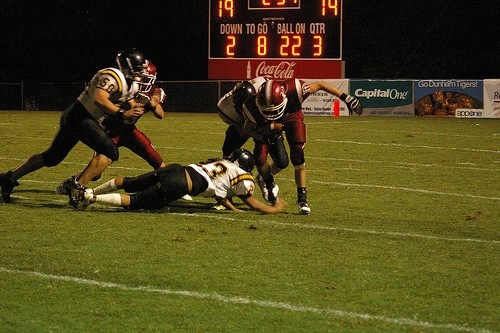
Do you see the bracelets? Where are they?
[151,107,156,112]
[340,93,348,101]
[117,108,125,118]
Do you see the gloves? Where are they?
[339,92,363,116]
[263,130,284,145]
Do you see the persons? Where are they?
[216,76,363,215]
[67,148,286,214]
[0,53,193,202]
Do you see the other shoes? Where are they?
[0,170,20,203]
[57,174,85,195]
[257,173,279,205]
[181,194,194,200]
[69,188,96,211]
[211,199,234,211]
[297,202,311,214]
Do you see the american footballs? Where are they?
[265,122,283,129]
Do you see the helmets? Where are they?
[133,59,157,92]
[228,148,255,172]
[115,48,146,82]
[256,80,288,120]
[232,80,259,124]
